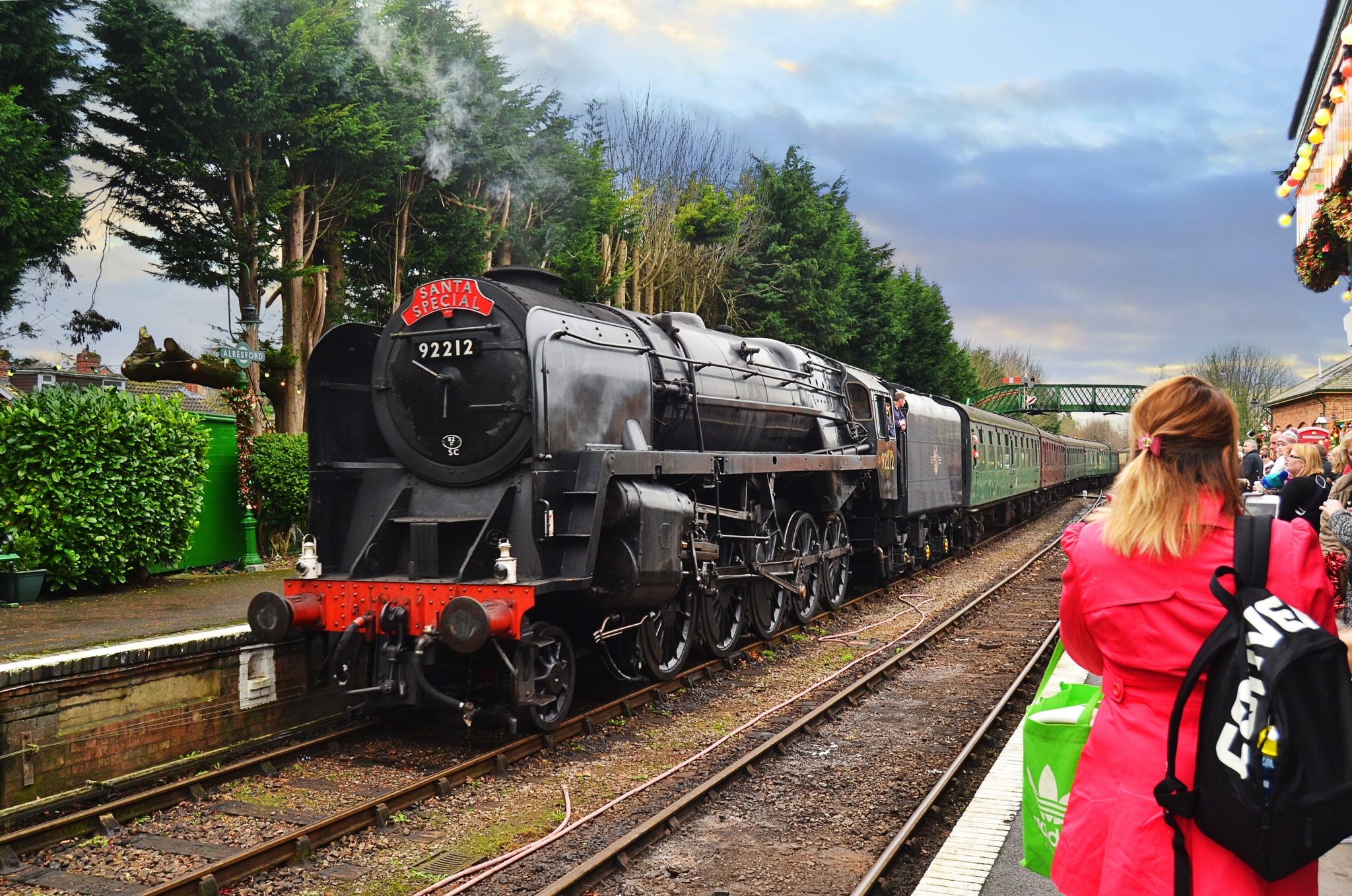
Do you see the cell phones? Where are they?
[1244,495,1281,519]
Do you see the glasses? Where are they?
[1289,454,1302,460]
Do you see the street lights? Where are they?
[1221,357,1230,397]
[1250,382,1267,444]
[228,262,265,570]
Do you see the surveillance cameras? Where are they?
[1247,430,1256,439]
[1021,376,1036,388]
[1250,397,1259,404]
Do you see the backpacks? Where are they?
[1152,516,1352,882]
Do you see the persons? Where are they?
[1253,428,1288,491]
[1270,427,1298,484]
[1264,443,1271,448]
[1261,451,1275,475]
[1259,447,1270,459]
[885,403,895,437]
[1315,430,1352,629]
[893,392,907,464]
[1261,471,1288,491]
[973,434,979,458]
[1278,443,1331,536]
[1237,440,1250,492]
[1050,375,1352,896]
[1239,439,1264,494]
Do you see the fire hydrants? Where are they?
[1331,398,1344,420]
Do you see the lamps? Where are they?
[1333,274,1351,300]
[1279,206,1296,226]
[1315,413,1329,424]
[1276,21,1352,198]
[1261,426,1271,431]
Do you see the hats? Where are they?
[1276,426,1298,456]
[1260,469,1288,489]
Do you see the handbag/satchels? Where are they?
[1020,679,1105,880]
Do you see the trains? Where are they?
[248,265,1119,736]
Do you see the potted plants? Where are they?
[0,529,47,605]
